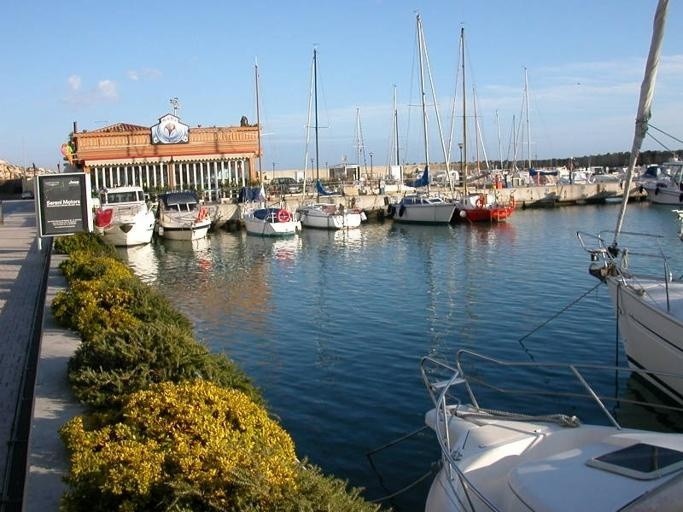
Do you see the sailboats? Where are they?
[577,0,682,413]
[387,12,459,224]
[441,25,517,223]
[298,45,368,230]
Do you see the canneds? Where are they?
[277,210,291,222]
[198,208,207,220]
[475,199,483,208]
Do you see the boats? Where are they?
[95,186,155,245]
[534,151,683,205]
[152,191,212,241]
[243,200,302,236]
[419,349,682,511]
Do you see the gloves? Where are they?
[534,151,683,205]
